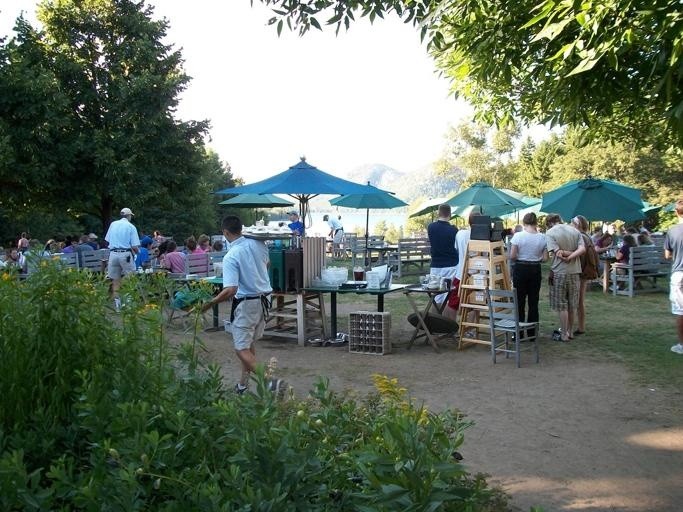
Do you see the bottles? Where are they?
[295,235,301,249]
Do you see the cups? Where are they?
[419,274,451,290]
[354,270,363,281]
[213,264,223,278]
[604,247,617,257]
[138,266,153,273]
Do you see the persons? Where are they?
[200,215,287,402]
[591,224,659,291]
[286,211,305,236]
[323,215,344,260]
[508,212,595,342]
[0,231,224,281]
[663,200,683,354]
[427,204,483,339]
[104,207,141,312]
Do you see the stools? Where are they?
[458,240,512,350]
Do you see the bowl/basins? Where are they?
[322,267,348,285]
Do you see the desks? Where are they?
[262,290,328,347]
[300,280,414,345]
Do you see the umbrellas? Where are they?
[217,192,295,226]
[408,175,663,231]
[219,155,396,236]
[329,179,410,248]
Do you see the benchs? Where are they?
[15,235,230,305]
[612,235,673,298]
[326,233,433,278]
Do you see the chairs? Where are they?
[485,286,539,368]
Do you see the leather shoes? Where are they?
[511,333,535,341]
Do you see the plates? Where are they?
[243,226,292,235]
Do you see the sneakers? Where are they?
[671,343,683,354]
[269,379,277,391]
[234,384,248,394]
[115,304,122,313]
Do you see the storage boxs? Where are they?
[470,216,504,242]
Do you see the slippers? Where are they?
[553,327,584,341]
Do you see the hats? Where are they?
[88,233,98,239]
[142,237,153,244]
[120,208,135,216]
